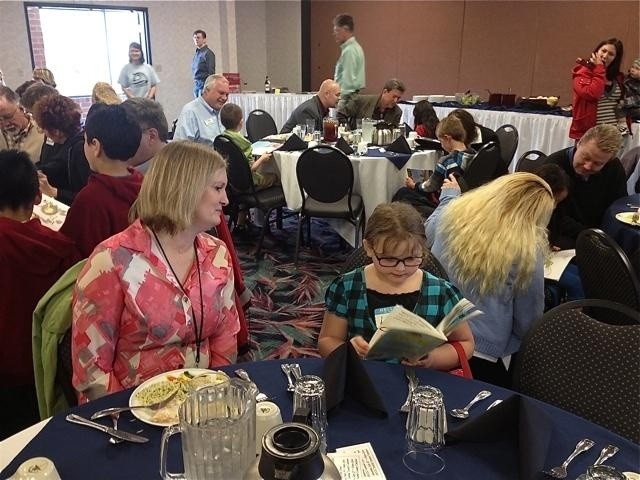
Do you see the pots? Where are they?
[488,93,518,110]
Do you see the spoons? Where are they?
[576,444,621,480]
[282,363,296,391]
[449,390,492,419]
[108,412,124,444]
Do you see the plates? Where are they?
[413,94,457,104]
[614,210,640,226]
[130,368,231,429]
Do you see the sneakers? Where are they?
[616,121,630,136]
[231,224,278,247]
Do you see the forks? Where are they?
[539,438,597,479]
[90,389,180,420]
[235,368,277,403]
[400,377,420,413]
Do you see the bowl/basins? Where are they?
[519,94,561,111]
[455,92,480,107]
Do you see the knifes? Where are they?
[66,414,150,443]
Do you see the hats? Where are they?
[33,68,55,87]
[633,57,640,66]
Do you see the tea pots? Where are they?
[242,407,342,480]
[158,377,259,480]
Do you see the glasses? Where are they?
[371,246,425,267]
[0,107,17,123]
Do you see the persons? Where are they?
[568,39,639,147]
[426,124,628,388]
[0,76,171,407]
[329,13,365,128]
[172,73,279,191]
[119,42,160,103]
[315,202,474,381]
[280,77,499,204]
[191,30,215,100]
[70,142,240,407]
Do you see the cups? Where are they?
[291,116,423,157]
[190,369,231,421]
[292,375,329,439]
[402,385,447,475]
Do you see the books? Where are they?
[363,298,484,366]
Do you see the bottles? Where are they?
[264,75,271,93]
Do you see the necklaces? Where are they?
[151,224,204,369]
